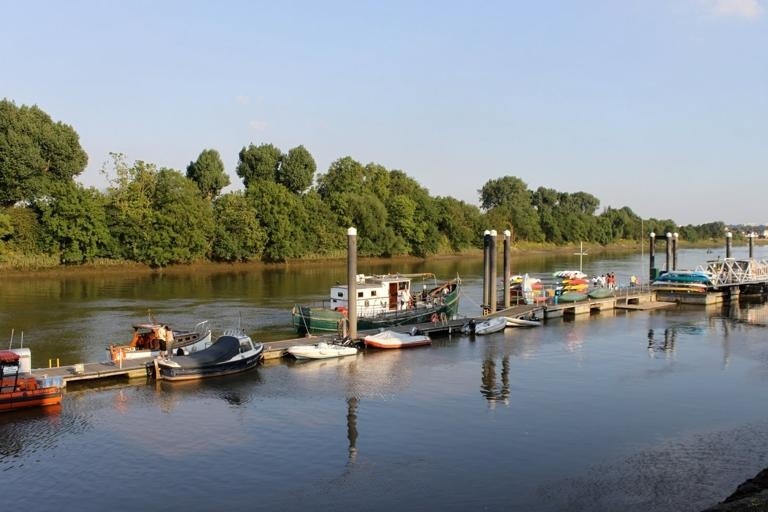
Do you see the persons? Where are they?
[140,337,145,350]
[592,271,616,291]
[630,274,637,287]
[400,284,413,310]
[155,323,175,358]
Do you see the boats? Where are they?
[496,275,549,305]
[292,272,461,335]
[145,334,264,382]
[547,269,613,302]
[284,343,359,360]
[106,319,211,363]
[0,348,62,413]
[460,316,507,336]
[360,331,432,350]
[500,313,542,328]
[650,257,755,292]
